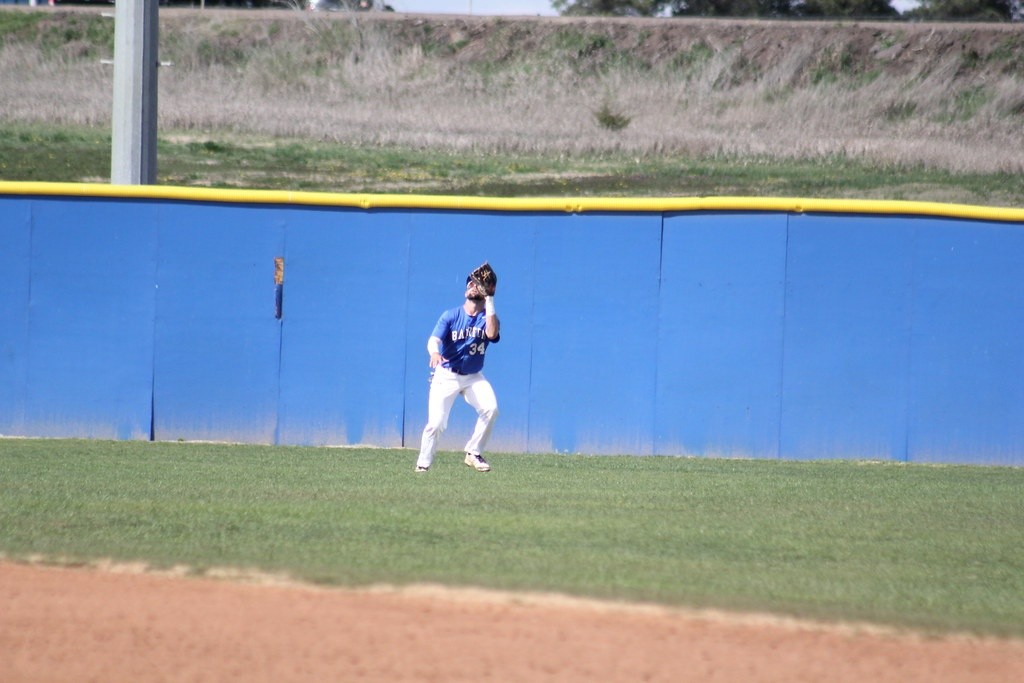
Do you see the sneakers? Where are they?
[414,466,428,472]
[464,453,490,472]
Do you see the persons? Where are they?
[414,261,501,472]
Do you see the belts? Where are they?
[449,367,467,375]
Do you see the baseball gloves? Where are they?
[470,262,498,296]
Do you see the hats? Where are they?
[466,275,473,286]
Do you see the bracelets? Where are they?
[427,341,438,356]
[486,296,496,316]
